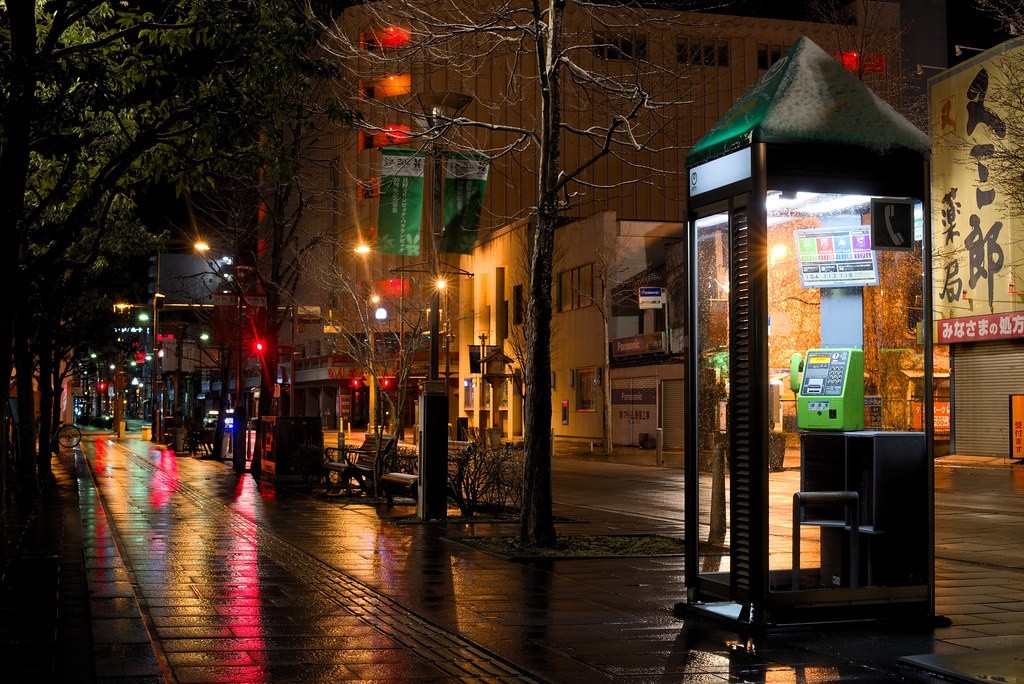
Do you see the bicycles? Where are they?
[34,412,82,448]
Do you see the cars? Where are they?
[74,406,82,415]
[208,409,234,428]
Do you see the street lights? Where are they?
[436,277,451,397]
[194,240,237,263]
[91,314,210,370]
[354,245,405,440]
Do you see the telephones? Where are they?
[788,348,865,430]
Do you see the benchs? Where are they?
[181,428,217,455]
[324,434,393,497]
[380,441,475,514]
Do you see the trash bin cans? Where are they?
[141,425,152,441]
[638,432,648,448]
[770,432,786,471]
[488,427,502,451]
[172,428,187,452]
[212,431,229,459]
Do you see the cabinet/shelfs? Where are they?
[799,429,927,590]
